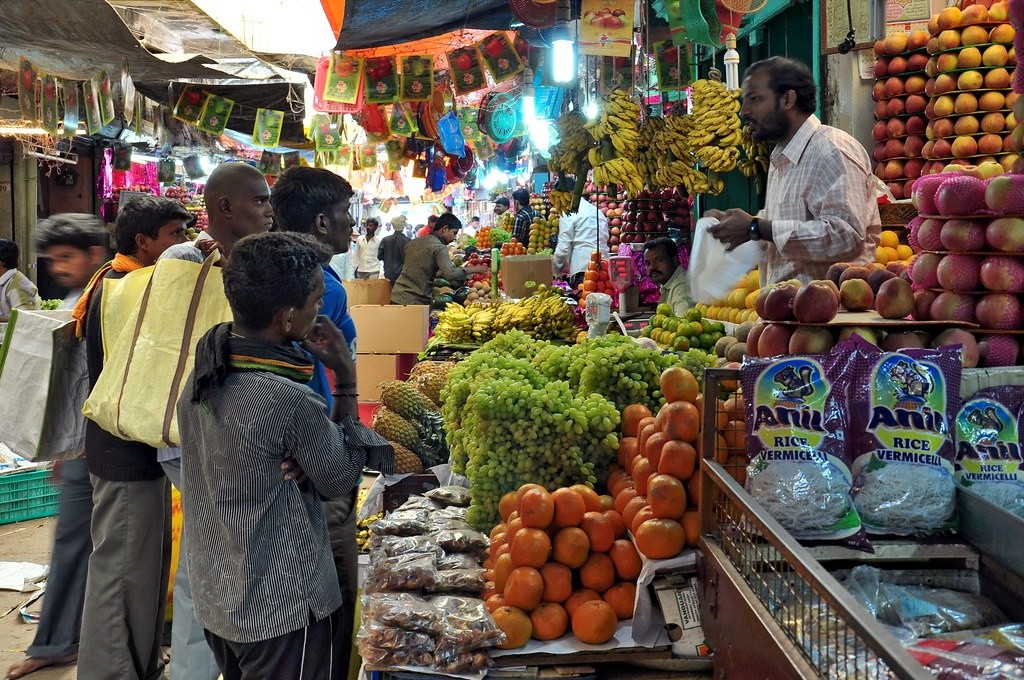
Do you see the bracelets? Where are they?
[334,381,357,389]
[332,393,359,396]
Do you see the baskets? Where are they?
[0,469,63,525]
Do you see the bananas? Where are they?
[427,288,583,346]
[547,79,772,215]
[355,487,384,553]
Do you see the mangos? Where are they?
[696,270,764,325]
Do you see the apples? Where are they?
[585,6,627,29]
[109,185,208,240]
[526,196,558,255]
[747,261,926,356]
[908,173,1024,368]
[871,0,1024,198]
[334,39,501,81]
[502,212,513,232]
[582,181,691,253]
[463,252,503,290]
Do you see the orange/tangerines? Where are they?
[607,365,754,559]
[480,481,643,648]
[641,302,726,353]
[474,225,491,249]
[578,251,616,308]
[875,230,915,265]
[500,236,527,257]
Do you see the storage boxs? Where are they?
[499,254,552,301]
[339,274,432,432]
[0,463,63,526]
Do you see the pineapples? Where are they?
[372,361,454,475]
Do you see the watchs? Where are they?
[750,218,760,241]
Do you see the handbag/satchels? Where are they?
[81,246,235,448]
[1,263,110,463]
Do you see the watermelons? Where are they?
[432,263,470,309]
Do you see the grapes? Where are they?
[40,298,63,309]
[488,228,512,243]
[439,328,722,529]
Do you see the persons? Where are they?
[1,57,882,680]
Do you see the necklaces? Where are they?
[228,329,246,339]
